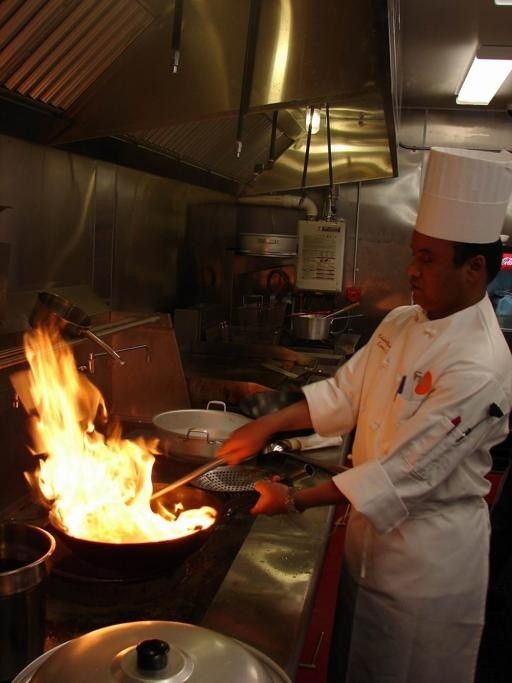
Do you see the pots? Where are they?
[27,287,129,371]
[2,519,55,665]
[54,478,302,556]
[154,399,255,460]
[286,301,359,339]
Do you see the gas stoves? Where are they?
[10,417,289,651]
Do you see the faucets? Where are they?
[265,268,294,305]
[89,343,151,364]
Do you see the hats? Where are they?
[413,147,512,245]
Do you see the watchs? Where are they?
[285,484,306,516]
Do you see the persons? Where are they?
[216,228,512,682]
